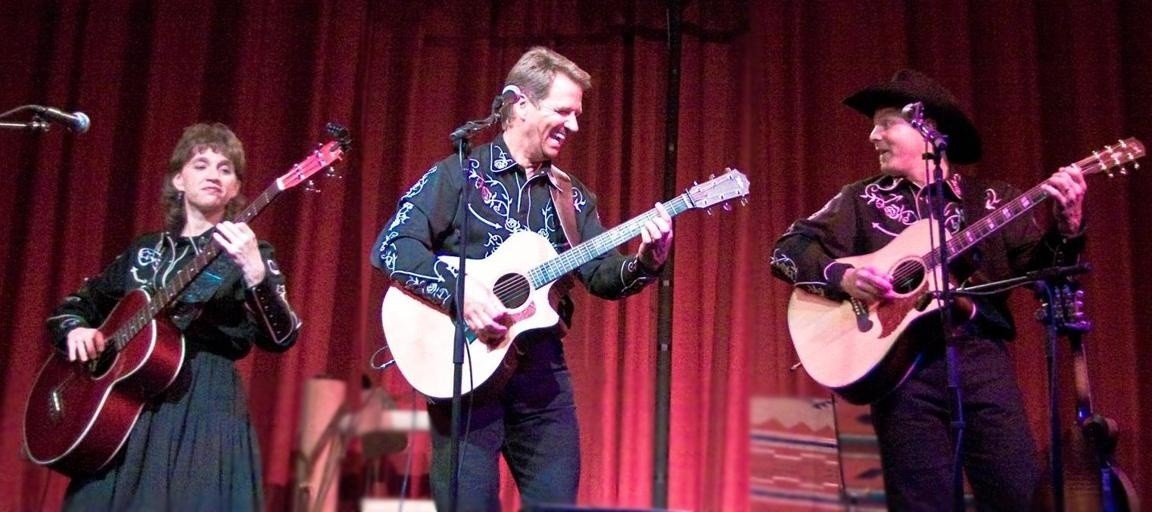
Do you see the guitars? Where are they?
[787,137,1146,406]
[380,167,751,410]
[20,122,353,477]
[1060,290,1139,512]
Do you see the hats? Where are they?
[841,69,983,165]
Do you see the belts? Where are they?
[943,318,991,340]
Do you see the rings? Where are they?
[1063,186,1071,194]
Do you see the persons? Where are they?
[769,67,1088,512]
[45,121,304,512]
[369,43,674,512]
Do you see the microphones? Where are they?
[445,94,507,141]
[900,98,947,158]
[29,98,92,137]
